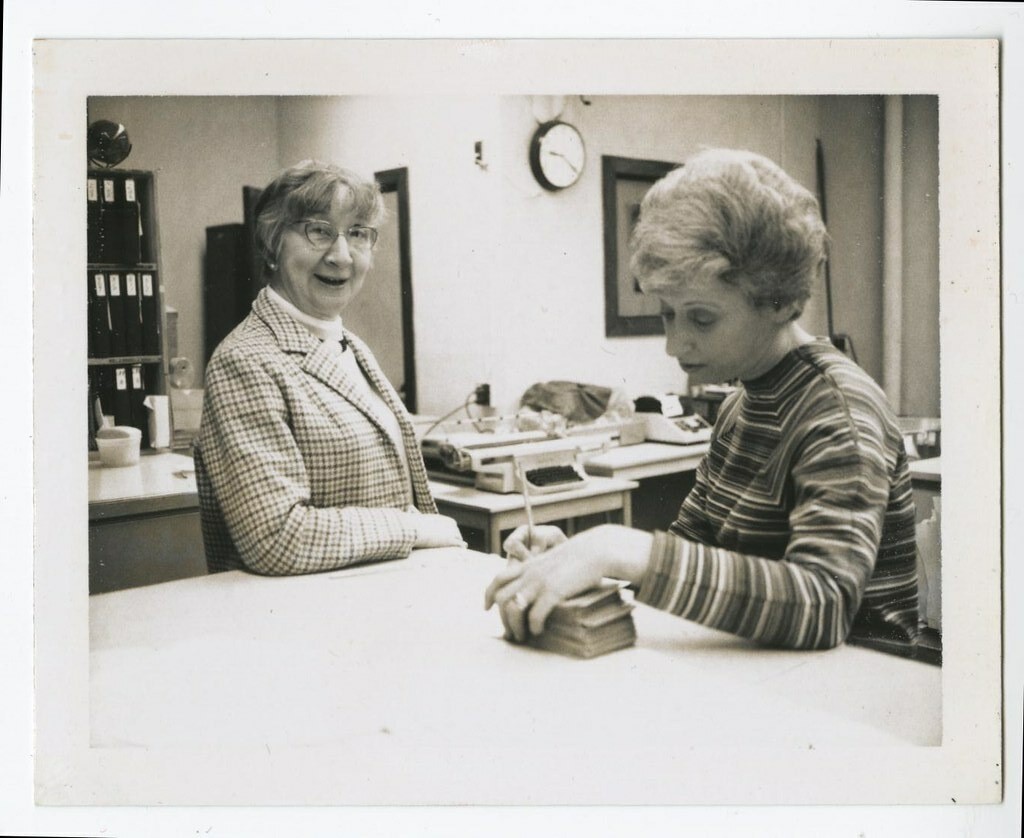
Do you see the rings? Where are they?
[513,594,527,611]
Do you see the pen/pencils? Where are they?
[516,459,538,551]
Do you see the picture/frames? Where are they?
[602,154,686,336]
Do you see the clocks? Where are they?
[530,119,585,191]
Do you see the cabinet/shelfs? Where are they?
[86,164,175,453]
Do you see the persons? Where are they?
[483,149,920,653]
[193,159,469,577]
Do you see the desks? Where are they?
[89,448,209,596]
[428,437,713,554]
[89,537,942,745]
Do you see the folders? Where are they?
[88,363,152,451]
[86,174,142,265]
[88,271,161,358]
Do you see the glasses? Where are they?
[287,219,378,249]
